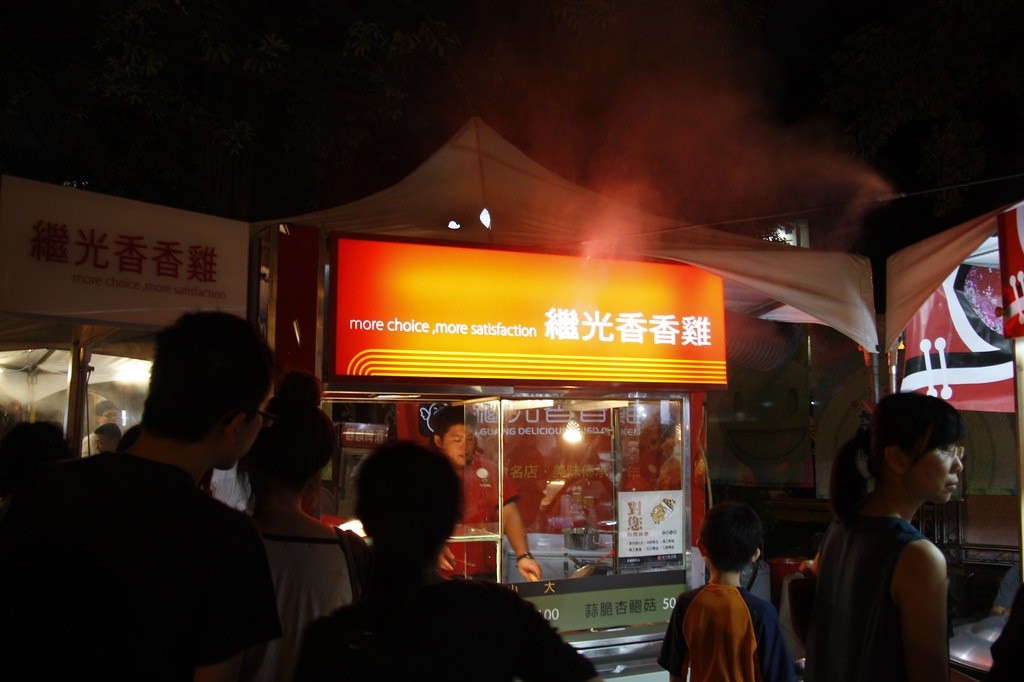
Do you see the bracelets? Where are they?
[517,553,533,562]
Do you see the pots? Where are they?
[562,527,618,551]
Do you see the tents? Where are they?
[69,104,881,411]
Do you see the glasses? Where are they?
[223,407,276,427]
[100,413,120,420]
[931,444,965,459]
[94,439,109,445]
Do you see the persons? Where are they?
[428,405,542,582]
[657,498,795,682]
[94,423,123,453]
[81,400,120,458]
[801,391,964,682]
[0,311,600,682]
